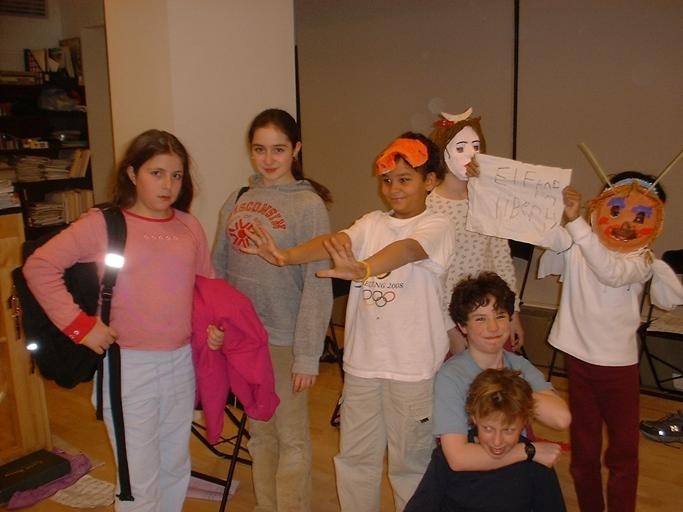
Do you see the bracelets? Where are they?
[521,439,538,465]
[352,260,372,284]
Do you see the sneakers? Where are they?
[639,408,682,443]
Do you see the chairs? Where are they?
[189,392,253,512]
[639,249,683,403]
[505,241,534,361]
[330,279,350,428]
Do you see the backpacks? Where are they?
[7,201,127,388]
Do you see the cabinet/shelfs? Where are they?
[0,28,117,468]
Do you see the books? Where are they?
[1,35,96,234]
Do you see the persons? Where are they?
[204,104,340,512]
[235,131,459,511]
[461,147,682,511]
[426,268,576,477]
[20,126,229,511]
[421,104,528,363]
[402,365,571,509]
